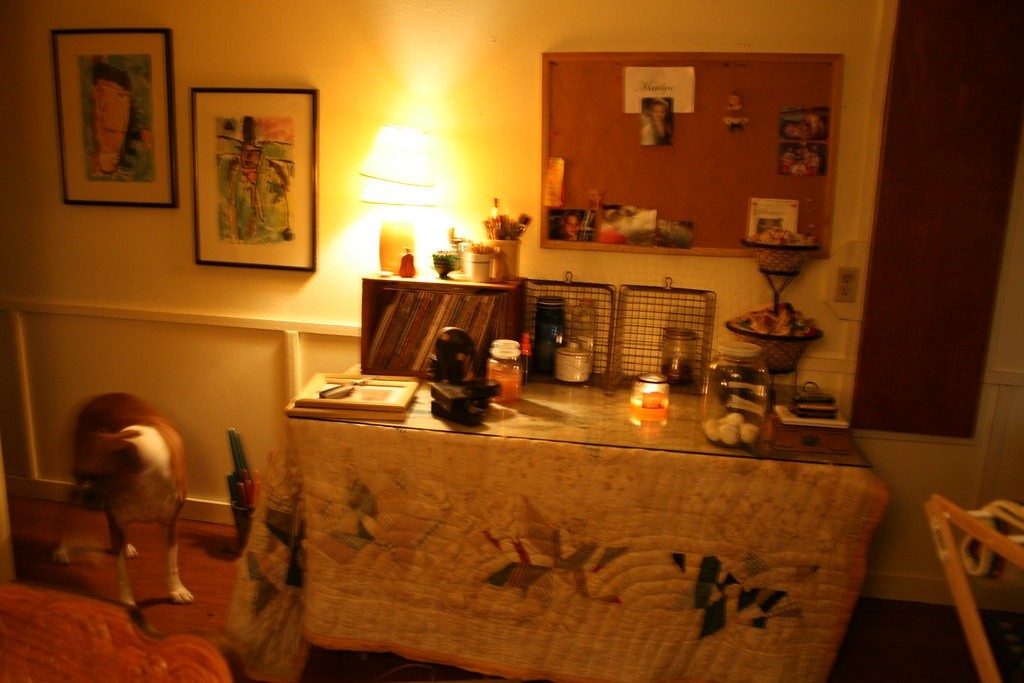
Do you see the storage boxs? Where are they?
[766,404,851,455]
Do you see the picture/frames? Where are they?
[190,87,319,272]
[50,26,182,209]
[293,373,420,413]
[284,388,408,421]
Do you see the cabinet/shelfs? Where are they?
[361,272,529,380]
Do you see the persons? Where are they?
[643,98,672,144]
[779,112,827,177]
[561,212,580,242]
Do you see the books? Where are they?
[368,289,504,376]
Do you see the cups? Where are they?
[431,250,463,280]
[554,338,594,383]
[630,372,670,423]
[481,238,523,282]
[464,252,491,283]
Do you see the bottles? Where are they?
[660,326,698,387]
[533,293,566,378]
[399,247,417,279]
[486,338,522,406]
[700,340,769,450]
[570,297,595,376]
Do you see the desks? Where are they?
[286,359,874,683]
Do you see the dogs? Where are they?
[46,392,194,611]
